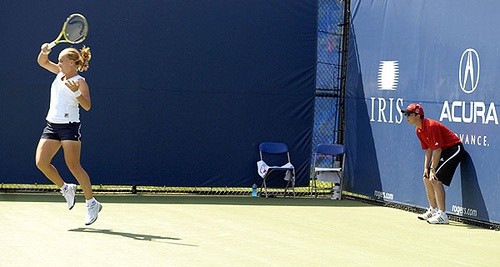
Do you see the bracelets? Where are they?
[40,50,52,55]
[71,89,82,99]
[429,167,434,169]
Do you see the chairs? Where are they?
[258,142,295,199]
[310,143,345,200]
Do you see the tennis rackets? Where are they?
[42,13,88,53]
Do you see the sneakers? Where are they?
[59,184,77,210]
[84,200,102,225]
[426,212,449,224]
[417,210,438,221]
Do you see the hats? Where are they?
[401,103,424,116]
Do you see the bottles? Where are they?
[334,183,340,200]
[252,183,257,196]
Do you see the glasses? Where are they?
[404,113,416,116]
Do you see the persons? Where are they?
[32,42,103,226]
[399,102,465,225]
